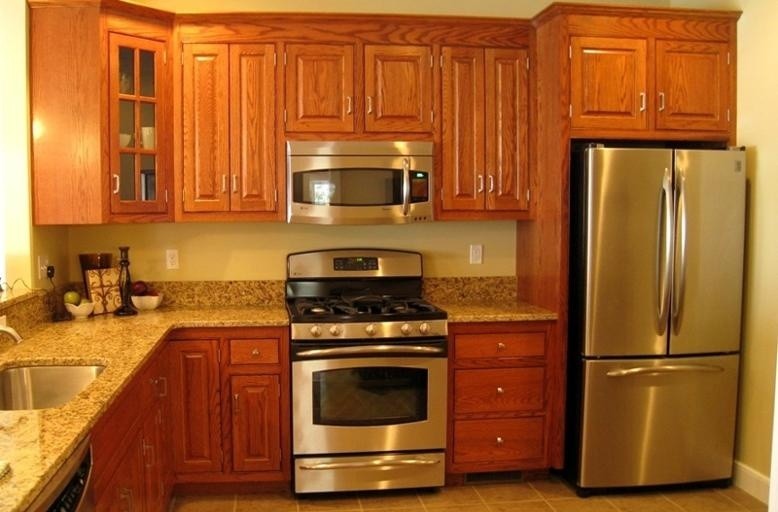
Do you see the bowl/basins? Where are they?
[64,303,96,320]
[130,294,164,312]
[118,133,132,148]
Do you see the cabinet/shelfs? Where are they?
[532,1,744,142]
[170,43,288,226]
[170,330,294,500]
[27,1,174,229]
[287,43,431,146]
[92,379,140,511]
[432,43,531,222]
[446,323,554,487]
[138,324,178,512]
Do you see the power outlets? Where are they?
[37,255,50,281]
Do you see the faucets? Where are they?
[1,326,23,346]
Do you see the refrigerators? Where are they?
[568,143,745,497]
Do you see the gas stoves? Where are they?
[292,295,441,316]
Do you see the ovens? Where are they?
[290,335,448,498]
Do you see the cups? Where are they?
[133,125,155,148]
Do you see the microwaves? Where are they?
[285,140,434,227]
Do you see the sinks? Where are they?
[1,364,106,412]
